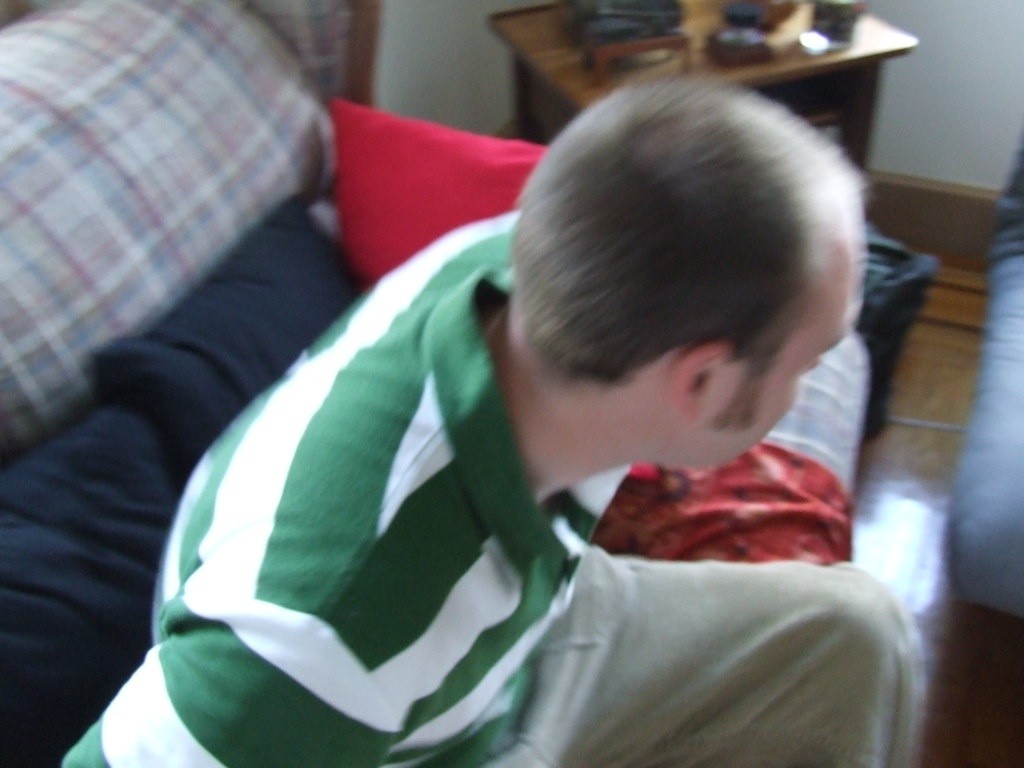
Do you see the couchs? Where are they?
[0,0,870,768]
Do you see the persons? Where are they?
[63,77,924,768]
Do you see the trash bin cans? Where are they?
[856,231,938,439]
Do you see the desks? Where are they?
[487,0,918,146]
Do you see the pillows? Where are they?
[87,192,359,469]
[0,405,185,768]
[329,101,544,282]
